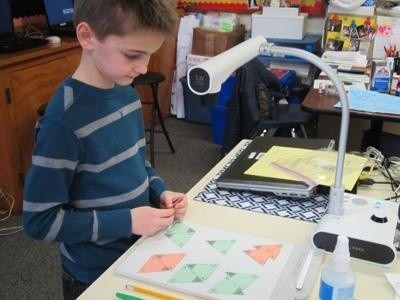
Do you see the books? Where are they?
[313,50,373,92]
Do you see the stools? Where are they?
[130,71,174,166]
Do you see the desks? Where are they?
[76,138,400,295]
[299,55,400,159]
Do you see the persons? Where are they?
[22,0,189,300]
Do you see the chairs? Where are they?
[233,56,316,139]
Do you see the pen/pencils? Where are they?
[384,45,399,57]
[115,285,181,300]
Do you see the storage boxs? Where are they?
[180,23,245,124]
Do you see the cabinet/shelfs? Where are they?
[0,30,174,212]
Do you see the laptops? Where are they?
[216,135,336,199]
